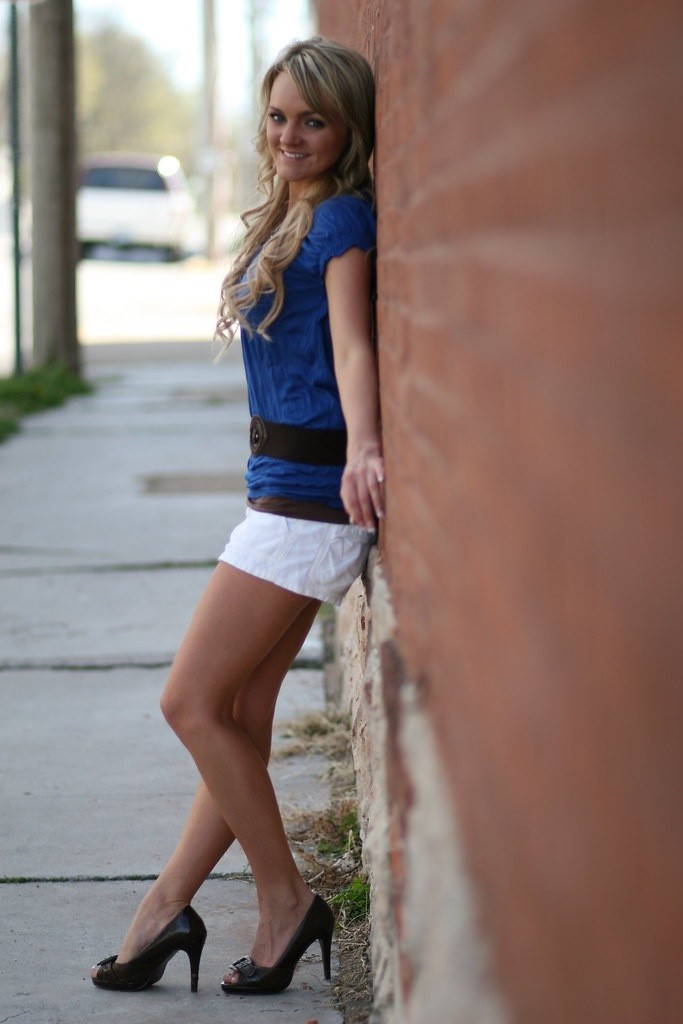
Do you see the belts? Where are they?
[247,415,352,469]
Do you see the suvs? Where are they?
[71,152,204,262]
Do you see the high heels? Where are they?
[93,907,209,993]
[218,893,336,994]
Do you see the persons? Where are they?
[88,36,386,996]
